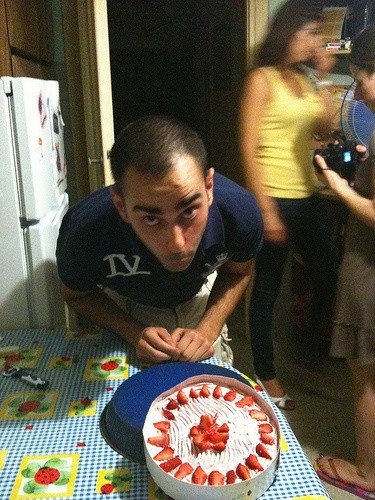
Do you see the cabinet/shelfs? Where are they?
[0,0,59,80]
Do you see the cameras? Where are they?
[313,130,360,171]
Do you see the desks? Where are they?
[0,324,334,500]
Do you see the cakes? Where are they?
[142,380,278,486]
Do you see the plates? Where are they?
[98,360,256,467]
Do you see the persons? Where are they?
[239,0,374,500]
[55,115,263,364]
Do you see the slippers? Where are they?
[313,452,375,500]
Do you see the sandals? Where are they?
[270,390,296,410]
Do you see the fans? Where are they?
[341,77,375,151]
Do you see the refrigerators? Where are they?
[1,74,71,335]
